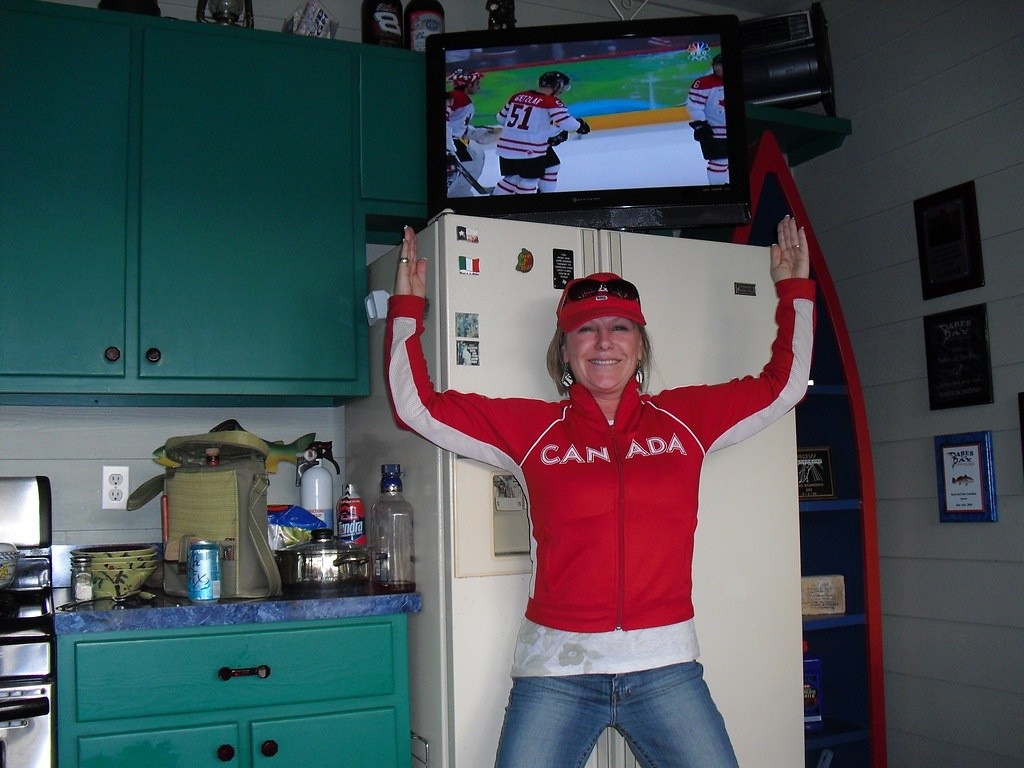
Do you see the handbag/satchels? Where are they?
[164,430,273,599]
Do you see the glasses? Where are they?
[561,278,641,310]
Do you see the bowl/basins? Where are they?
[70,546,159,599]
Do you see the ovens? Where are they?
[0,679,58,767]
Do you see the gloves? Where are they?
[574,118,590,135]
[462,124,499,145]
[547,129,568,147]
[689,119,715,144]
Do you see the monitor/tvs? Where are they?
[426,13,752,232]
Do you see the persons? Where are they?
[490,71,591,195]
[383,214,816,768]
[446,106,461,197]
[446,68,503,197]
[686,53,731,185]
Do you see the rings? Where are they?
[792,244,800,249]
[399,258,408,263]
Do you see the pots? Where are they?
[0,544,26,591]
[273,530,387,599]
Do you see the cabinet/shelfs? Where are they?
[0,0,431,408]
[730,128,889,768]
[53,586,422,768]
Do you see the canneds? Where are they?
[186,540,222,604]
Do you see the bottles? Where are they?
[73,556,93,606]
[337,484,367,546]
[368,463,416,592]
[206,448,219,466]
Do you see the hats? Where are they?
[556,272,647,333]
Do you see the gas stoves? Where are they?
[0,476,53,678]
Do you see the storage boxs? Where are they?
[802,575,845,615]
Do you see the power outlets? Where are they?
[102,465,130,511]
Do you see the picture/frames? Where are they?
[933,430,1000,523]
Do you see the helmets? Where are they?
[712,53,722,77]
[452,66,484,86]
[539,71,572,95]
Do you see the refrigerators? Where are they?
[343,206,805,767]
